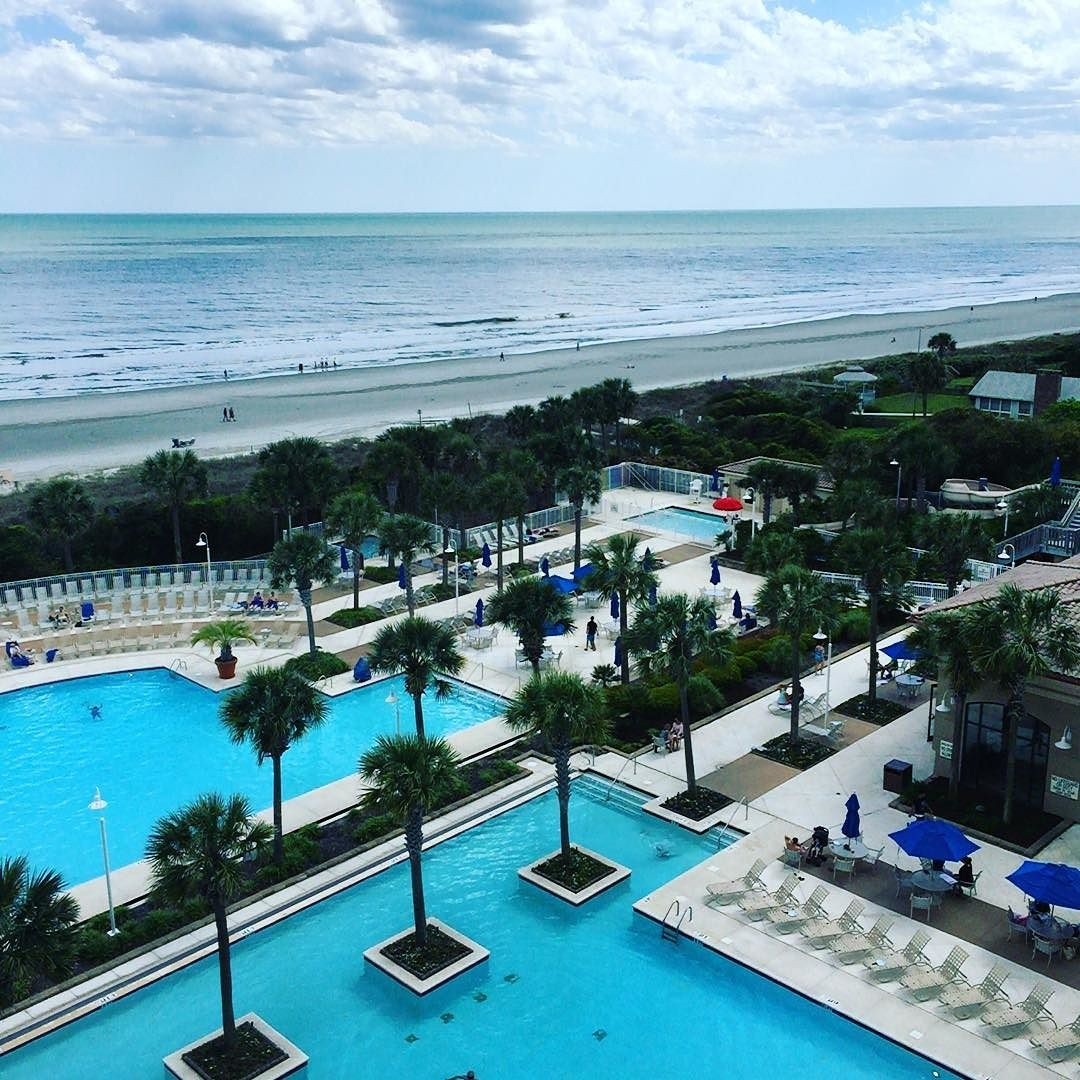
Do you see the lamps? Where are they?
[935,690,951,713]
[1055,725,1072,750]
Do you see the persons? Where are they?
[660,718,684,753]
[814,640,825,675]
[952,856,973,896]
[790,838,823,858]
[10,644,25,659]
[55,606,71,626]
[1029,897,1050,921]
[584,616,598,651]
[249,591,278,610]
[875,652,898,679]
[692,475,703,504]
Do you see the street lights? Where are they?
[742,487,756,542]
[195,532,216,619]
[889,458,904,526]
[444,538,459,619]
[385,684,400,737]
[87,785,121,937]
[996,492,1009,539]
[811,622,833,732]
[997,544,1016,570]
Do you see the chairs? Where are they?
[705,815,1080,1063]
[863,657,880,678]
[893,659,908,677]
[330,521,765,673]
[803,688,834,715]
[0,567,290,672]
[800,721,842,743]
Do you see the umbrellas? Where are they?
[878,641,926,680]
[1048,456,1061,491]
[1005,860,1080,920]
[713,497,743,526]
[341,543,350,578]
[399,561,407,607]
[475,542,743,683]
[842,791,860,848]
[888,819,981,878]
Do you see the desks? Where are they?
[895,674,925,695]
[912,868,956,892]
[828,837,869,859]
[1027,914,1075,943]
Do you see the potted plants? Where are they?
[190,617,258,679]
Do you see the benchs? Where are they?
[769,695,812,717]
